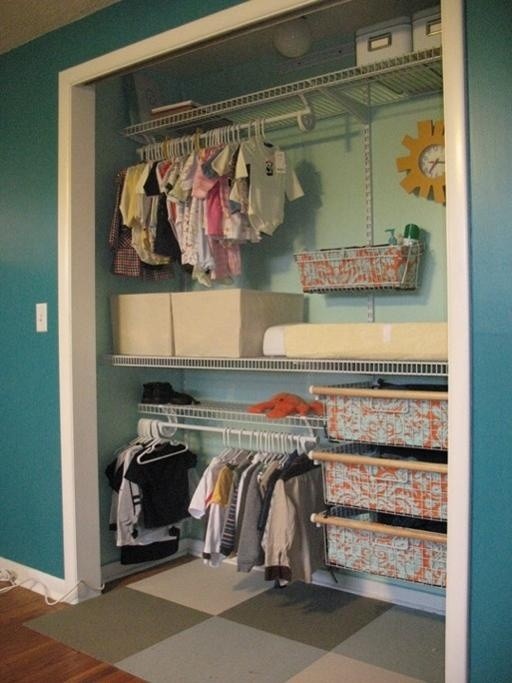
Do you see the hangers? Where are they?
[136,115,273,162]
[133,420,323,472]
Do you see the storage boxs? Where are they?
[413,5,445,73]
[110,293,171,358]
[172,288,306,356]
[352,12,441,94]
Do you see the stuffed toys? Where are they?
[247,392,325,418]
